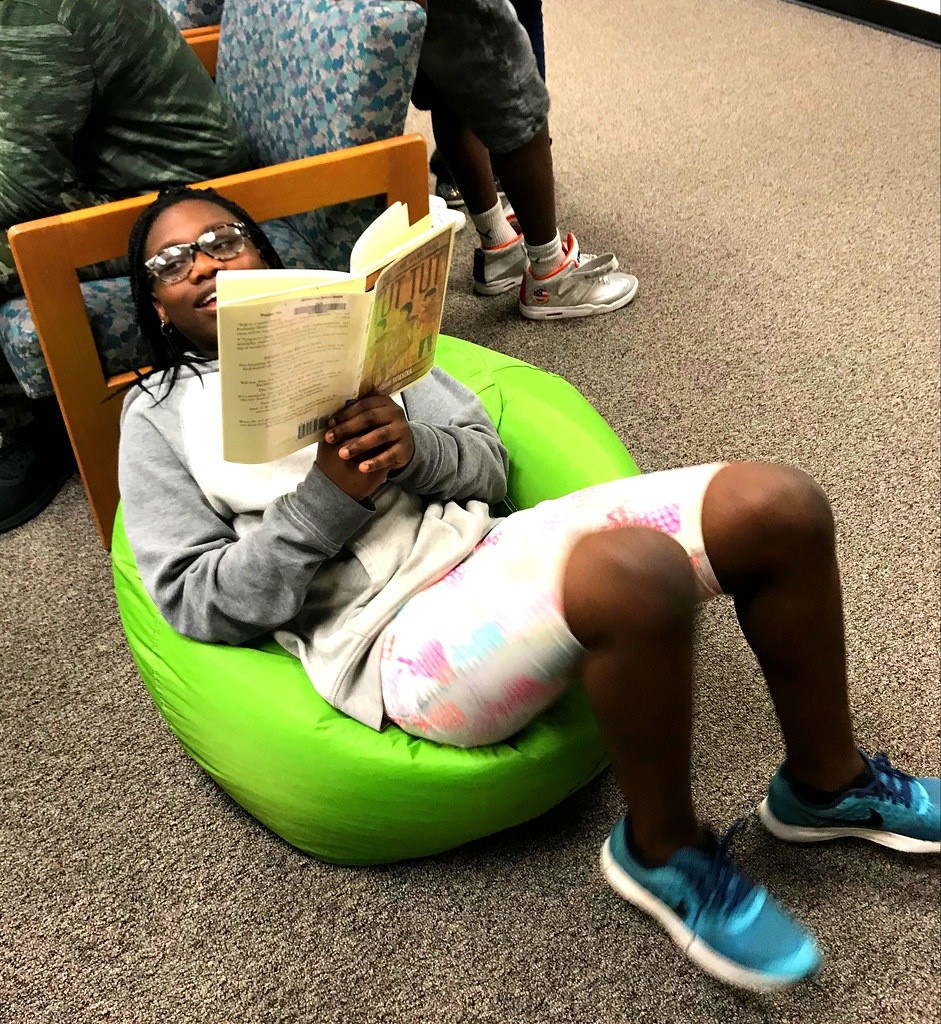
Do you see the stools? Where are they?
[111,341,648,867]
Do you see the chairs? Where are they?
[0,0,428,539]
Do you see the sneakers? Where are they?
[519,233,640,319]
[760,750,941,851]
[599,816,823,991]
[473,215,530,295]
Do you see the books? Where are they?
[216,202,455,462]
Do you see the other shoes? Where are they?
[435,177,505,206]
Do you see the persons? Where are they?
[99,186,941,989]
[0,0,639,536]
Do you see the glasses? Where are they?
[144,222,248,283]
